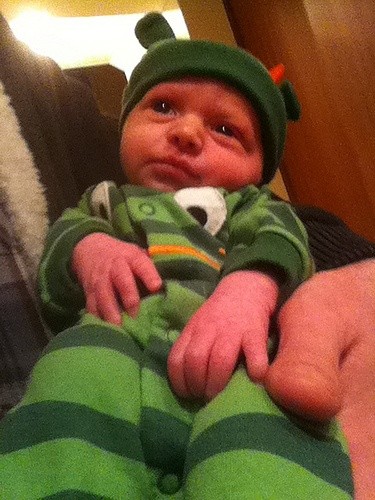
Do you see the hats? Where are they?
[118,11,300,186]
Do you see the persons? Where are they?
[0,12,354,500]
[0,12,375,500]
